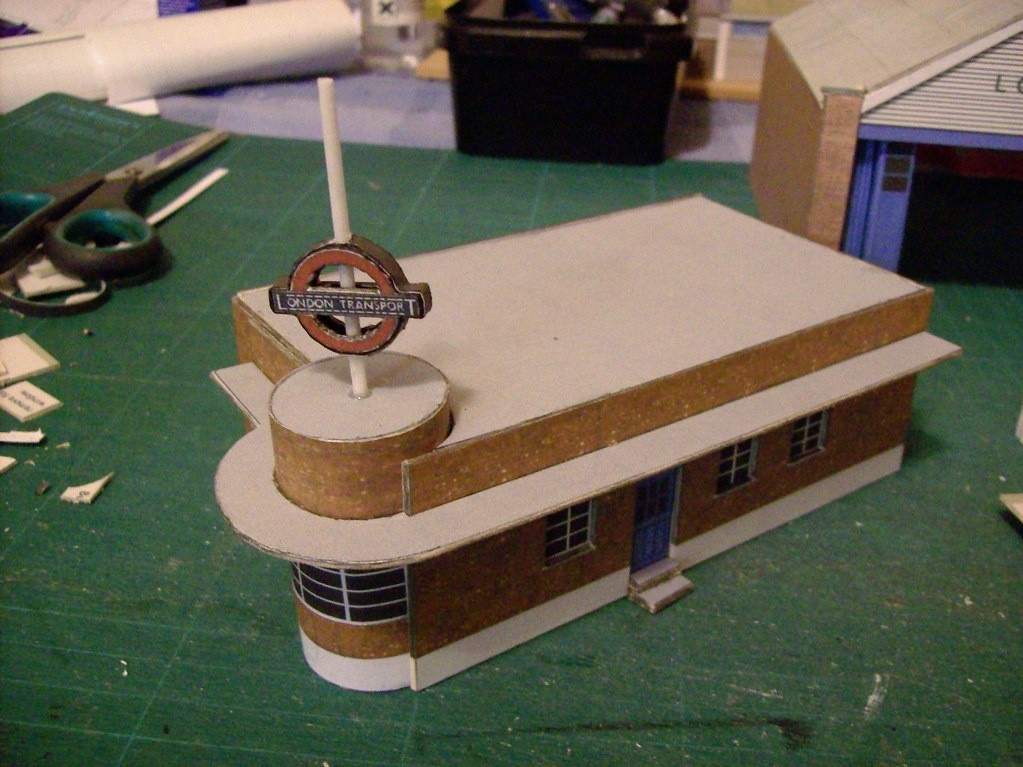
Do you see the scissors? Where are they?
[0,129,231,281]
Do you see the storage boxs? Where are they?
[436,1,694,166]
